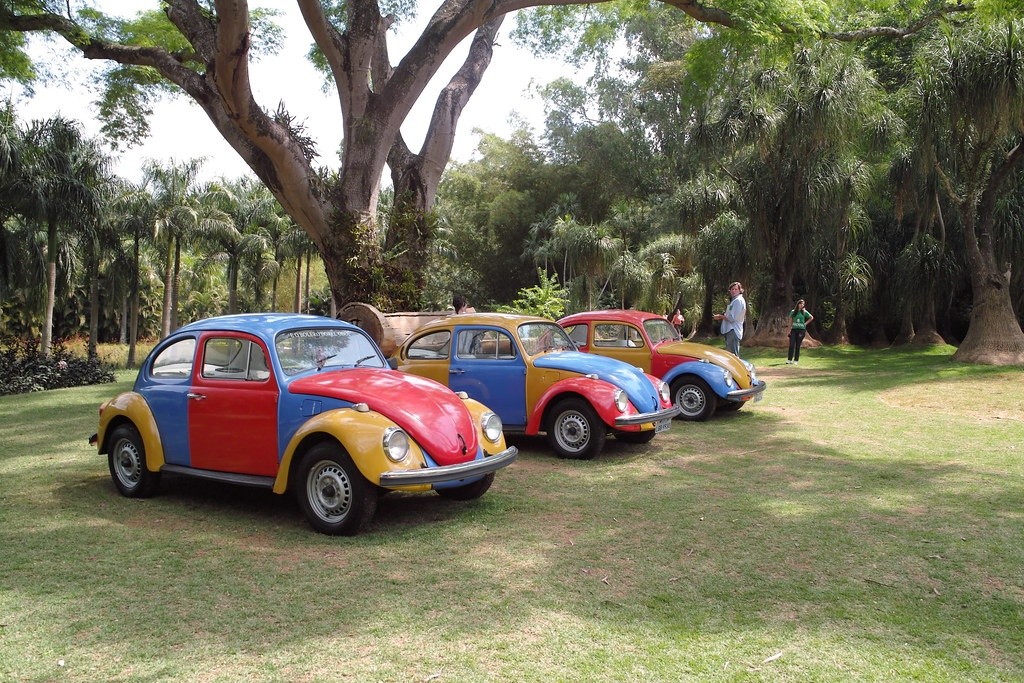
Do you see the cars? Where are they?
[89,311,520,538]
[530,309,766,421]
[383,312,680,459]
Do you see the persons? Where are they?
[671,309,684,335]
[712,283,746,356]
[787,300,813,365]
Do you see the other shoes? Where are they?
[793,361,798,365]
[786,360,791,364]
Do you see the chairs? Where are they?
[616,328,636,348]
